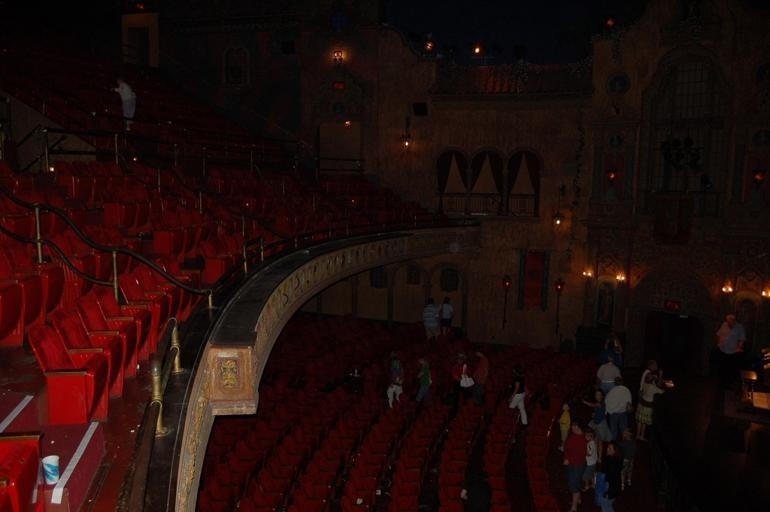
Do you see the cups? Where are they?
[43,454,61,485]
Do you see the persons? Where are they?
[107,78,137,134]
[714,313,746,392]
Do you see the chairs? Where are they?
[0,147,439,511]
[207,322,598,510]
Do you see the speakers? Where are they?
[407,264,420,284]
[282,41,294,54]
[371,267,387,287]
[439,269,458,292]
[413,102,427,116]
